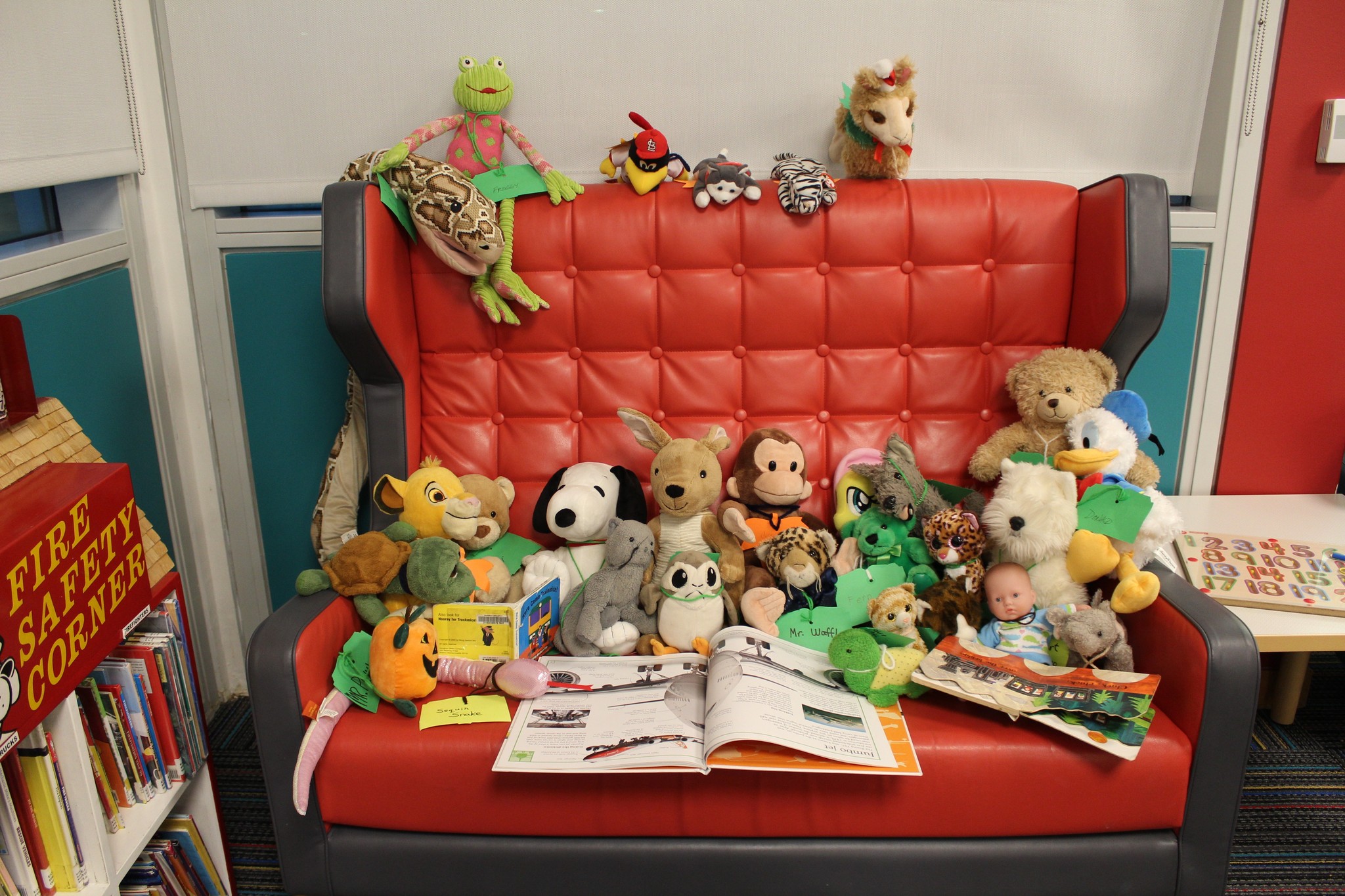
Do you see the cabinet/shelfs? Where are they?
[2,572,235,896]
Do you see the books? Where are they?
[0,596,222,896]
[430,580,1158,775]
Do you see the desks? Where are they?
[1160,493,1345,725]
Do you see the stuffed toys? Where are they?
[338,58,917,325]
[296,347,1182,676]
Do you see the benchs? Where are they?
[245,175,1263,896]
